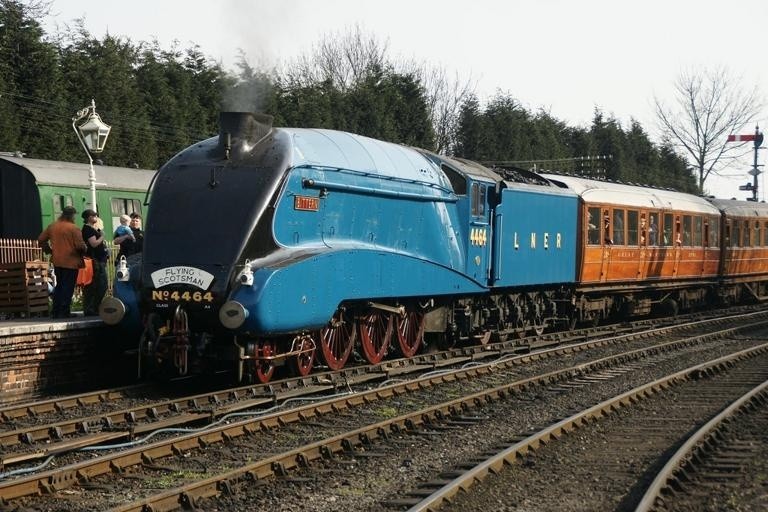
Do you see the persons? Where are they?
[113,211,144,265]
[92,211,110,260]
[38,204,88,319]
[81,209,109,317]
[112,213,136,268]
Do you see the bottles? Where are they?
[82,210,98,218]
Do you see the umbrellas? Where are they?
[53,311,78,318]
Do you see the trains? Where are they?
[134,110,768,388]
[1,151,160,311]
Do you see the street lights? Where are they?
[70,97,112,229]
